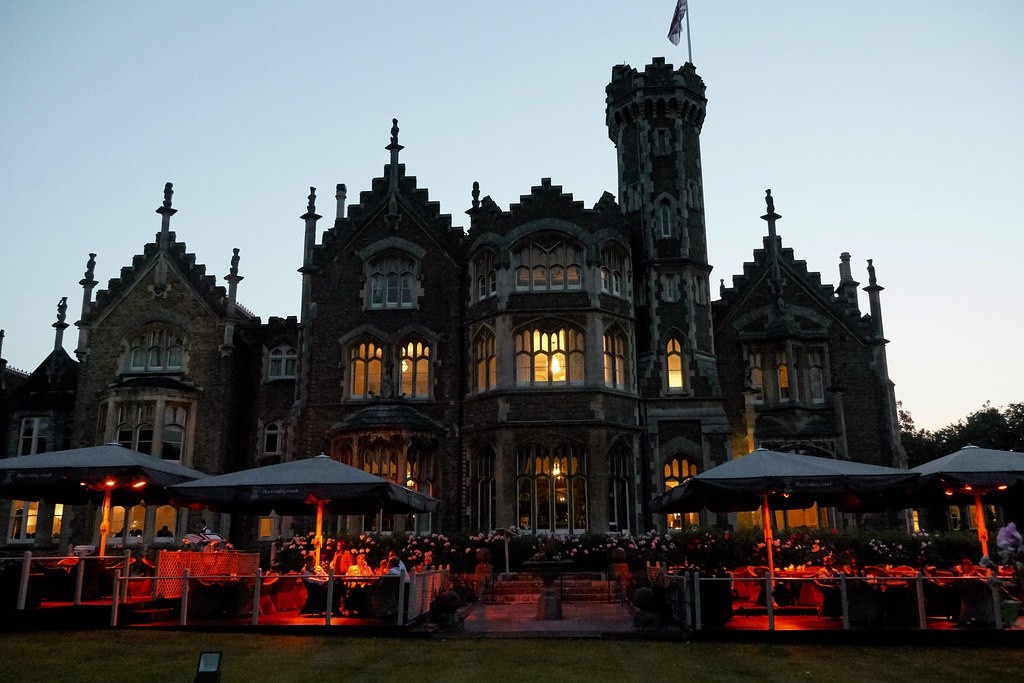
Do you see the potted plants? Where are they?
[474,550,494,576]
[609,551,630,577]
[533,562,562,622]
[436,590,460,630]
[629,588,661,630]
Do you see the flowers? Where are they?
[526,542,570,562]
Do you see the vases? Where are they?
[522,560,574,576]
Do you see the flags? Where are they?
[666,0,686,46]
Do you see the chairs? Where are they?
[189,572,400,615]
[28,558,157,599]
[733,566,1016,605]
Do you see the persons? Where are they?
[330,537,356,576]
[114,524,143,537]
[814,555,843,617]
[996,522,1024,564]
[610,548,634,595]
[377,550,411,586]
[157,526,173,537]
[961,559,986,578]
[198,519,212,534]
[342,553,375,607]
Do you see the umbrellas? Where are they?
[649,445,922,578]
[0,443,215,561]
[169,450,438,569]
[907,442,1024,556]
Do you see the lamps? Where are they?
[195,650,223,683]
